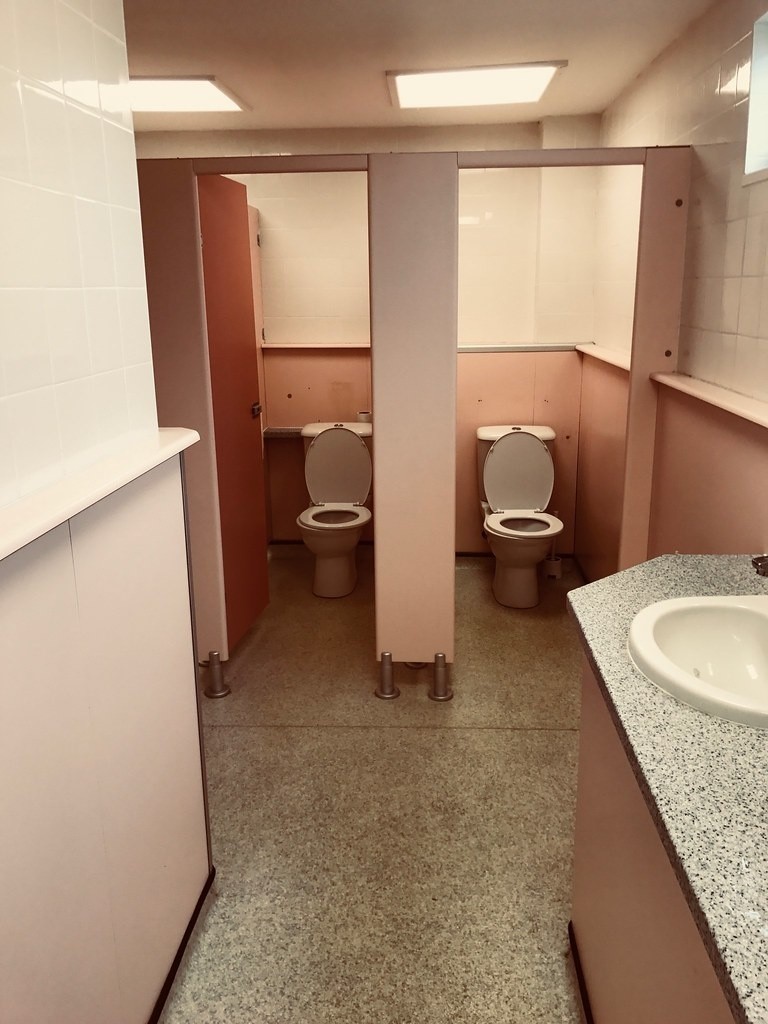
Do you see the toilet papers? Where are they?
[356,410,372,423]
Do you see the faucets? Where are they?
[752,555,768,576]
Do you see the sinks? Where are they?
[625,593,768,731]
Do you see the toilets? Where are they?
[476,425,557,609]
[296,422,373,598]
